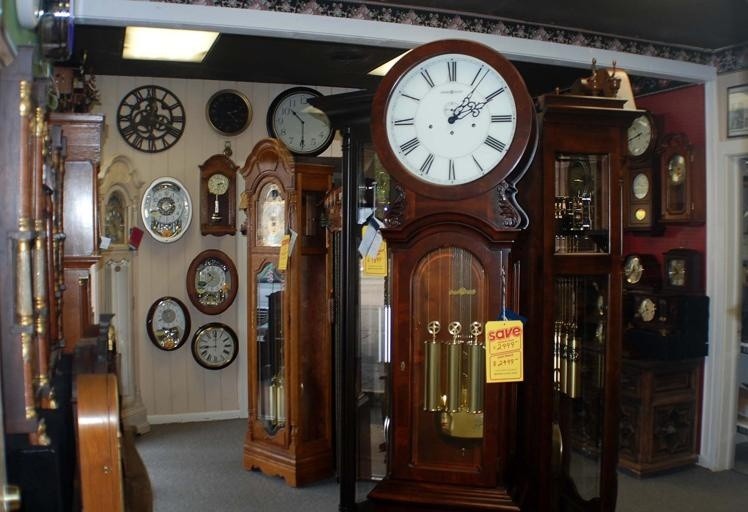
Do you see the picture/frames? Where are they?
[725,83,748,138]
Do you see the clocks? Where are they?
[198,139,240,238]
[192,322,240,370]
[206,88,254,136]
[99,153,152,436]
[662,130,696,223]
[364,37,540,512]
[147,295,192,352]
[663,249,701,287]
[530,57,645,512]
[187,249,238,314]
[266,85,334,158]
[141,176,193,244]
[239,136,334,489]
[631,294,659,327]
[621,254,660,289]
[626,108,658,230]
